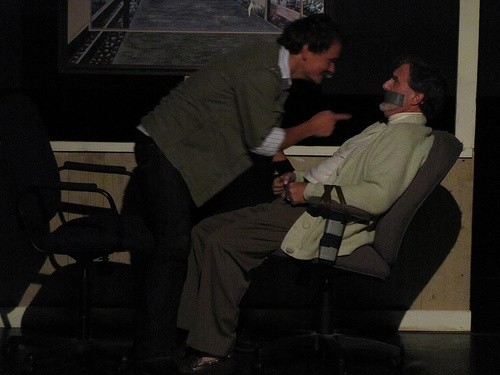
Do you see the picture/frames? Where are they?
[56,0,330,76]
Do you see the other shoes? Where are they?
[136,351,181,374]
[180,352,238,373]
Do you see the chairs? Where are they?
[248,130,462,375]
[0,93,154,375]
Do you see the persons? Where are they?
[174,52,457,375]
[135,13,352,375]
